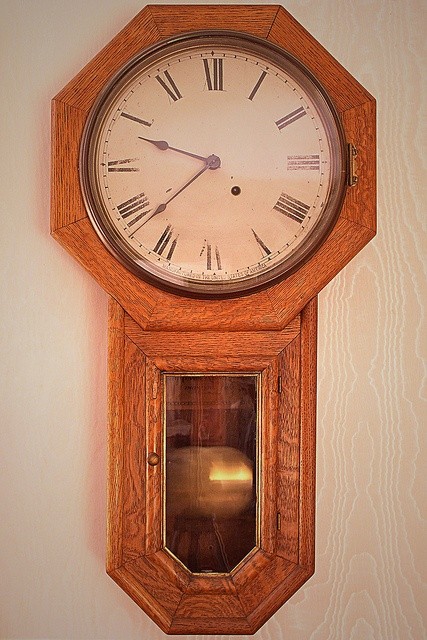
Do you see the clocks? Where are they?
[78,27,349,299]
